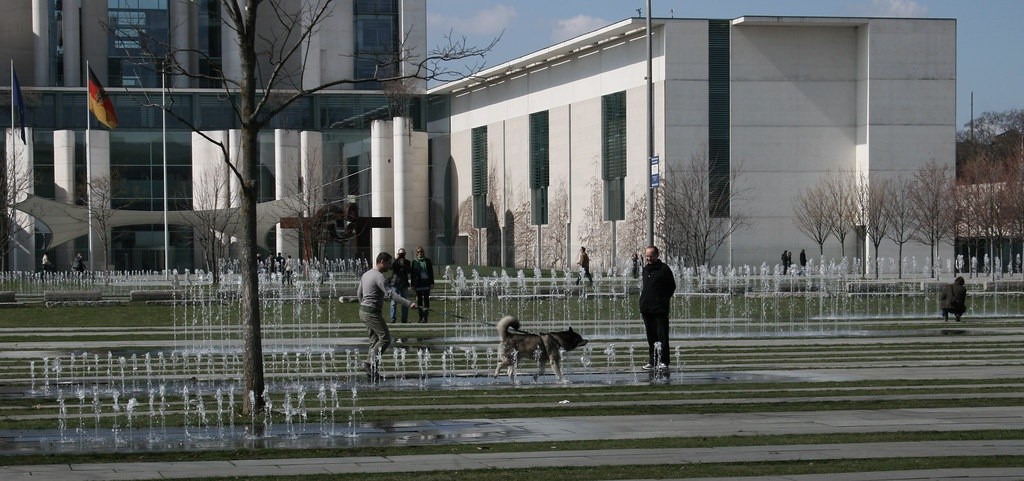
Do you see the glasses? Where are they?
[417,251,422,253]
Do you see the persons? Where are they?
[269,253,293,284]
[639,246,676,369]
[799,249,807,276]
[575,247,592,285]
[357,252,418,379]
[40,251,52,282]
[941,277,966,321]
[781,250,792,275]
[391,247,434,323]
[632,254,643,279]
[72,253,88,283]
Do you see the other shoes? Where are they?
[360,362,382,383]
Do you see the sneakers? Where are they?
[642,364,654,370]
[655,363,668,370]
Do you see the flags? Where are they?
[88,64,119,130]
[13,65,26,143]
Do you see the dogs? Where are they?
[493,315,588,384]
